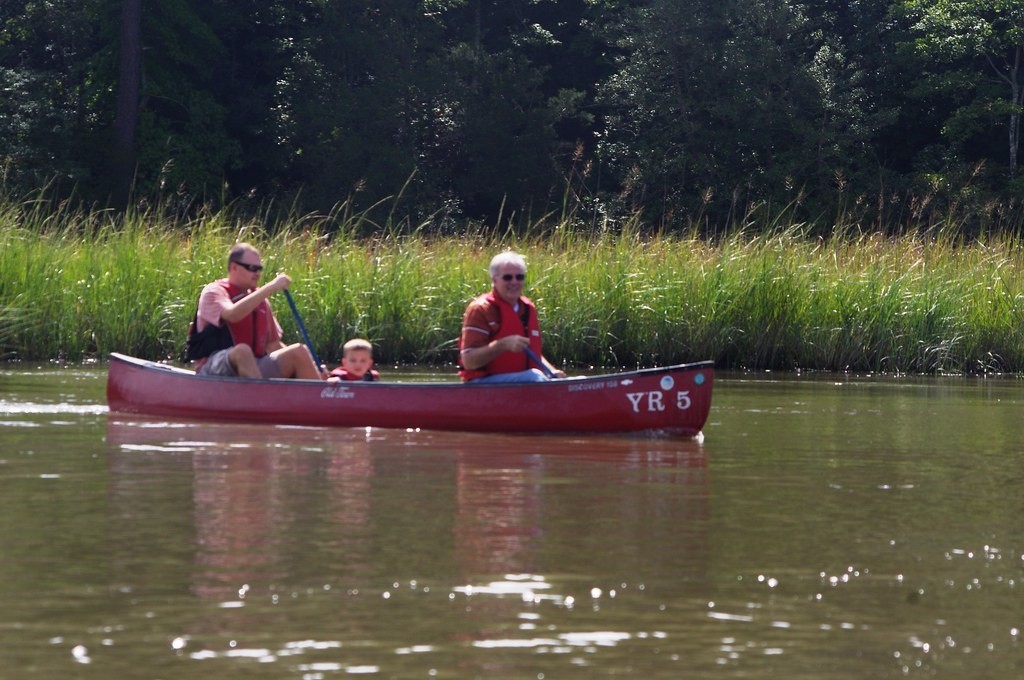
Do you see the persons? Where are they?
[327,338,380,381]
[458,251,567,383]
[187,242,321,379]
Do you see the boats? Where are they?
[104,348,713,440]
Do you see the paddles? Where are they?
[283,285,330,379]
[521,342,556,378]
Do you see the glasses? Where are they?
[231,259,263,273]
[494,273,525,282]
